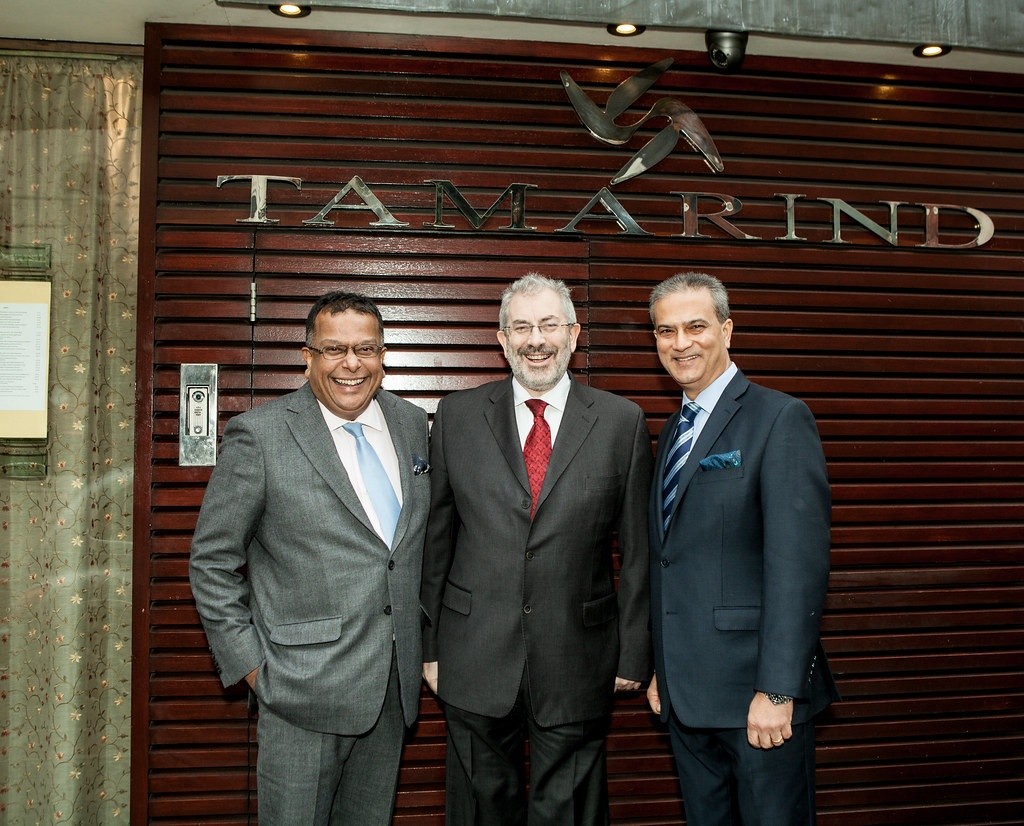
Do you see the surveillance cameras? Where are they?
[705,29,749,71]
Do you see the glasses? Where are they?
[307,344,383,360]
[501,322,579,333]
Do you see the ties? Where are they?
[662,402,702,539]
[521,399,551,522]
[341,422,402,551]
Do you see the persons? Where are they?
[189,291,431,824]
[417,272,656,826]
[642,270,836,826]
